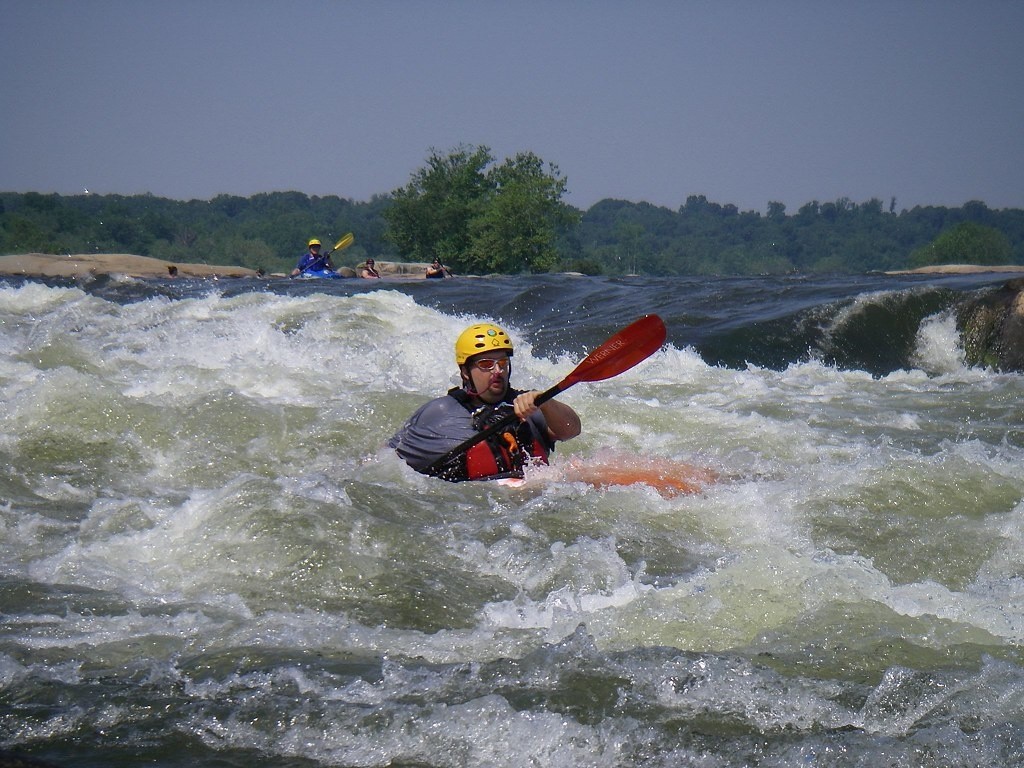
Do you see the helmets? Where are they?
[455,323,514,365]
[308,239,322,246]
[366,259,374,265]
[432,257,441,264]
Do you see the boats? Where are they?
[289,269,344,280]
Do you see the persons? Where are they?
[169,266,178,282]
[362,257,379,278]
[426,257,445,279]
[291,239,341,279]
[387,324,580,483]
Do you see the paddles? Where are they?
[414,314,670,477]
[435,256,453,277]
[290,232,354,278]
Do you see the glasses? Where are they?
[465,356,510,369]
[311,245,321,248]
